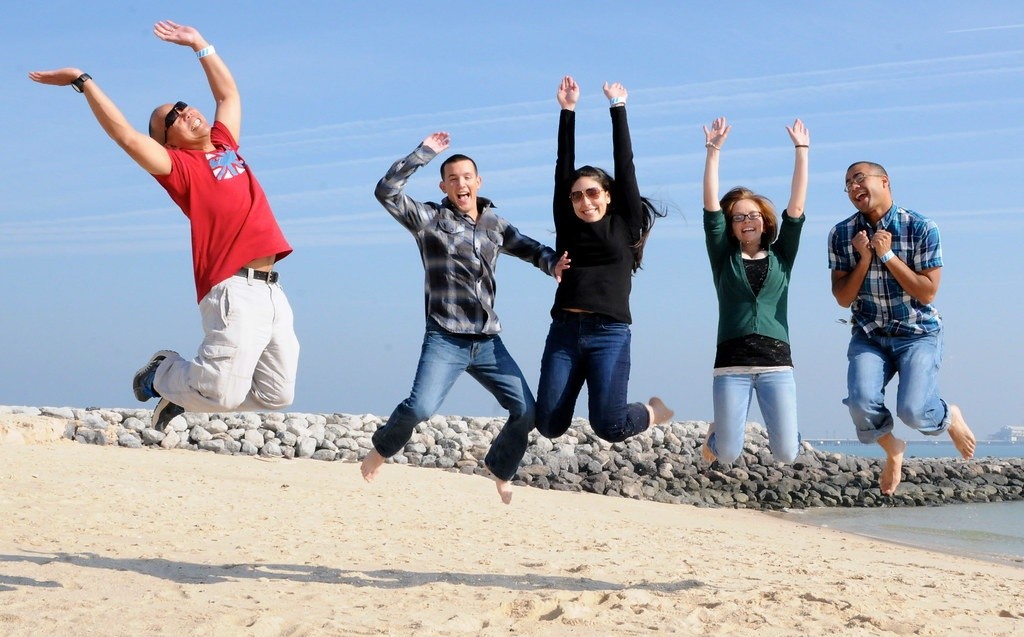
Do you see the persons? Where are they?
[28,21,300,431]
[829,163,976,494]
[703,117,810,465]
[360,131,571,505]
[535,76,674,443]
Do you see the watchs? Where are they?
[71,74,92,92]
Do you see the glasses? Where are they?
[569,187,605,201]
[843,173,882,192]
[164,101,188,145]
[731,211,765,222]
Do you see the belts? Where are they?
[235,268,278,283]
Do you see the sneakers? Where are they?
[151,397,184,431]
[133,350,180,402]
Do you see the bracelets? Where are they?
[795,145,808,148]
[195,44,215,58]
[705,142,720,150]
[880,249,894,263]
[609,96,627,105]
